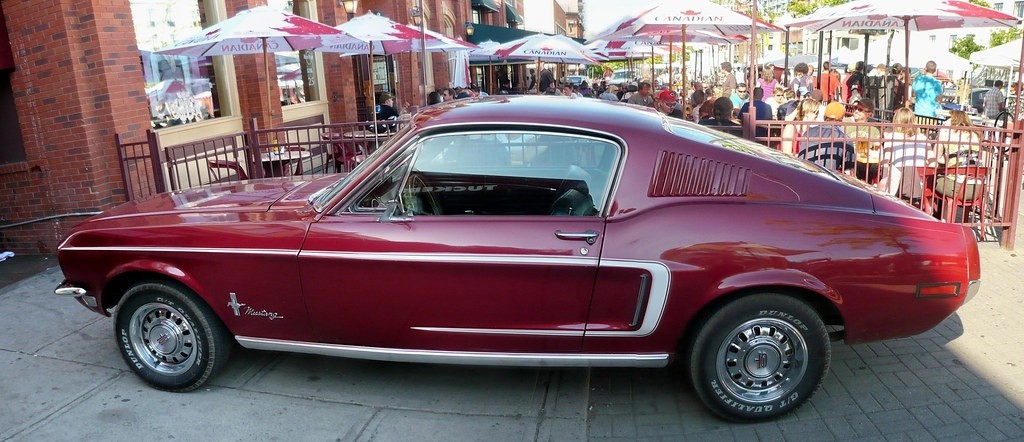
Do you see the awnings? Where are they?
[506,5,523,23]
[472,0,499,13]
[466,20,587,63]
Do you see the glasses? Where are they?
[738,90,747,94]
[444,94,450,97]
[853,105,873,114]
[613,90,619,92]
[775,94,783,97]
[662,101,676,107]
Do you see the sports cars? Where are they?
[54,94,983,425]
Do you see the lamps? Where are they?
[338,0,361,14]
[464,21,474,36]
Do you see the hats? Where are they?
[892,63,903,73]
[824,102,847,119]
[379,91,396,103]
[854,98,876,110]
[658,90,677,102]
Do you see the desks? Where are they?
[252,151,313,177]
[344,130,383,156]
[937,110,1013,156]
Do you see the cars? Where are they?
[562,62,695,88]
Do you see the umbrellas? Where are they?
[339,24,484,107]
[584,0,789,118]
[146,79,211,98]
[479,34,599,95]
[546,35,609,87]
[280,68,302,80]
[333,12,481,126]
[785,0,1023,107]
[154,6,370,149]
[276,54,300,66]
[582,30,750,91]
[590,44,691,92]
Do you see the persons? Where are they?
[369,63,1024,231]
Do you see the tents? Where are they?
[960,39,1024,117]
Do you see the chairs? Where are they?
[935,167,992,242]
[548,189,585,215]
[321,132,341,173]
[332,136,356,173]
[284,147,305,176]
[871,164,938,217]
[207,160,248,180]
